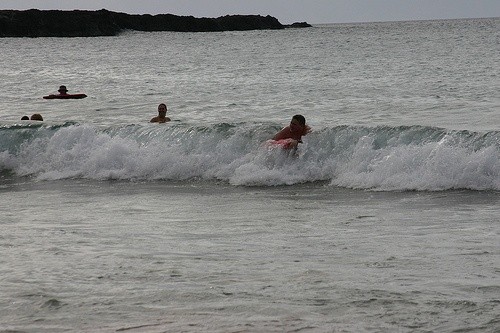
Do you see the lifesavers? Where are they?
[42,93,87,99]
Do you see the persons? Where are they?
[272,114,313,147]
[149,104,172,123]
[58,86,68,95]
